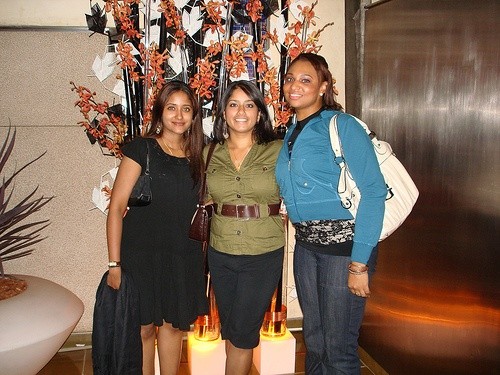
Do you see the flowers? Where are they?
[69,0,338,334]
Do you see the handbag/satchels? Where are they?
[187,205,210,242]
[328,114,420,243]
[127,138,152,207]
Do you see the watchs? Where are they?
[108,261,120,268]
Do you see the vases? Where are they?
[192,269,220,341]
[262,213,290,336]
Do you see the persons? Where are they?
[108,80,211,375]
[278,52,387,375]
[203,80,283,375]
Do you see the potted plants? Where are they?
[0,117,85,375]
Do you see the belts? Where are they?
[212,201,279,218]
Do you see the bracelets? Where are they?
[348,263,367,275]
